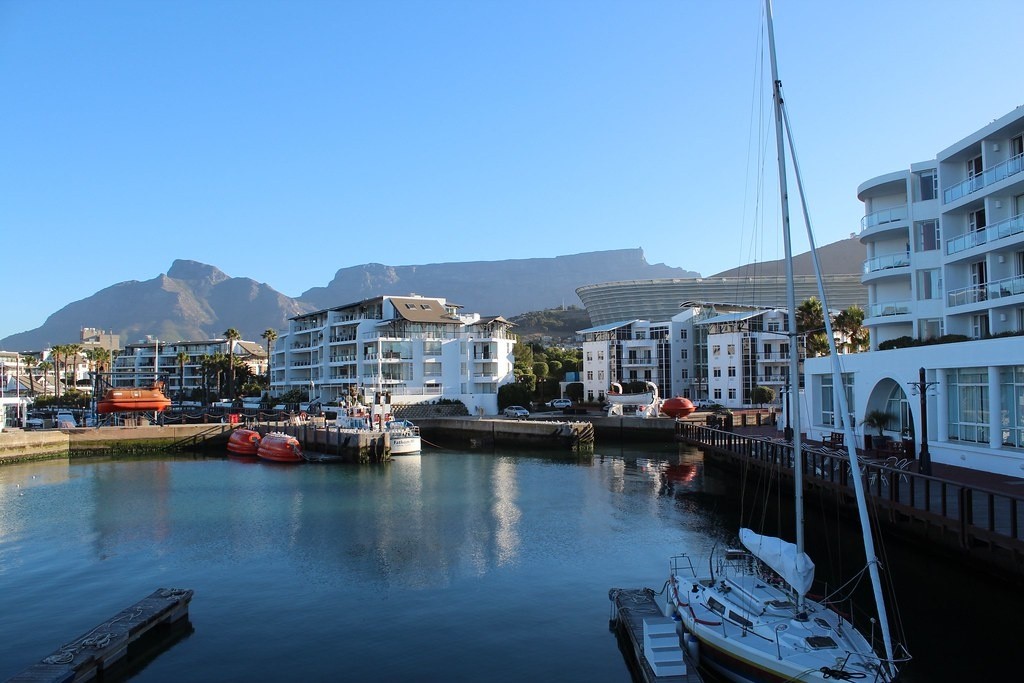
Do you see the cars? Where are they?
[692,399,719,410]
[26,410,101,428]
[503,406,529,418]
[545,399,572,409]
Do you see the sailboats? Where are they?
[668,0,913,682]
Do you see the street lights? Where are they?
[780,380,792,439]
[907,367,940,476]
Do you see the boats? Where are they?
[336,333,421,455]
